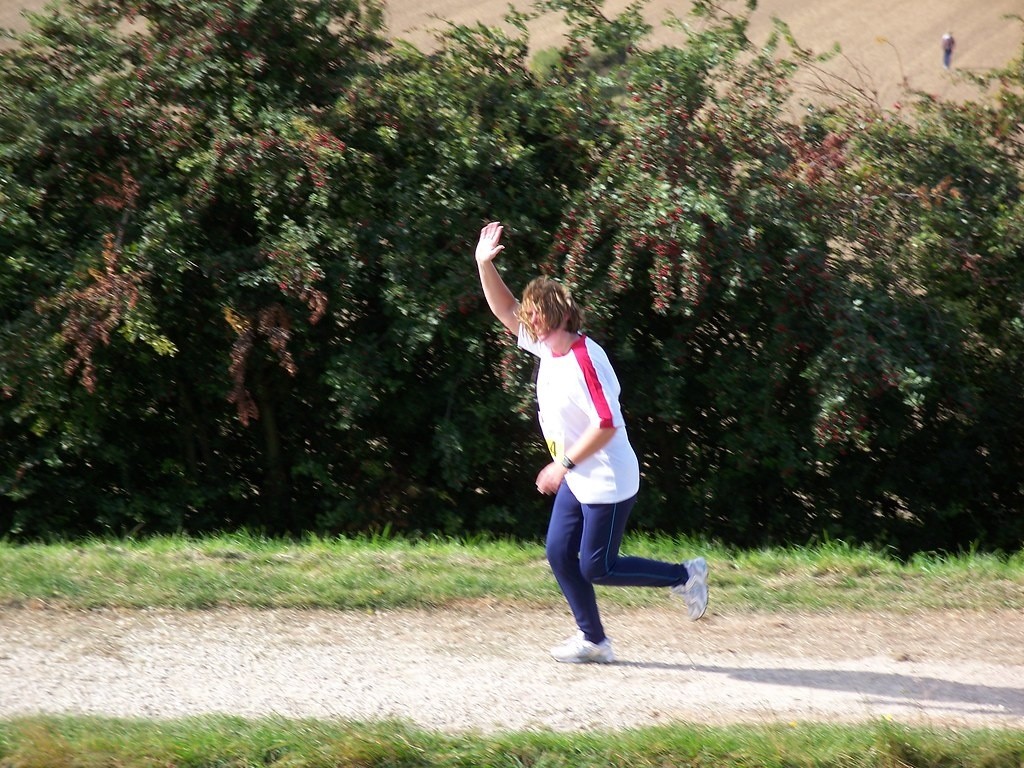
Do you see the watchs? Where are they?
[561,454,576,469]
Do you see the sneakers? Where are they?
[669,558,708,621]
[550,629,613,663]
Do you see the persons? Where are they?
[475,221,710,663]
[940,31,956,70]
